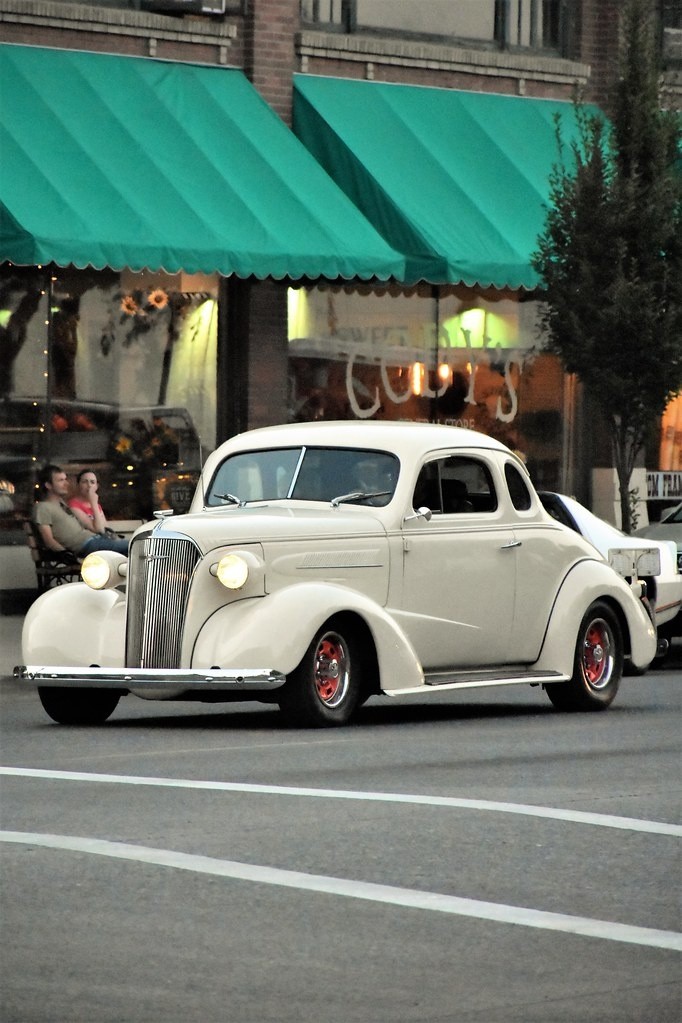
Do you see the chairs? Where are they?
[422,478,475,512]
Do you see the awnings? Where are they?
[0,42,403,305]
[290,69,682,296]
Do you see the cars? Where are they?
[0,398,213,527]
[8,416,674,729]
[469,488,682,668]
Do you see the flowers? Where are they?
[99,286,169,358]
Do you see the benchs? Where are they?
[24,517,148,596]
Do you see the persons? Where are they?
[68,469,108,534]
[287,370,384,424]
[33,465,132,558]
